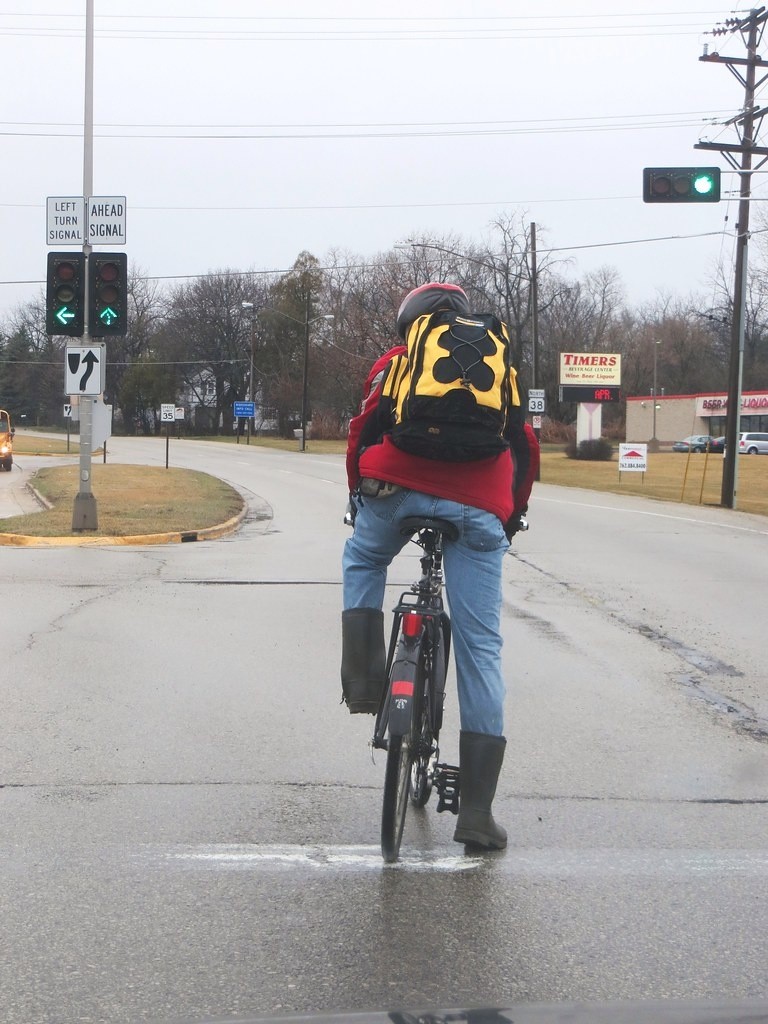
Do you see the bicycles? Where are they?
[343,508,530,860]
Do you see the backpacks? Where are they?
[379,307,522,464]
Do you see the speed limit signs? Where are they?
[160,403,176,422]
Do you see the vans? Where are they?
[738,432,768,456]
[0,410,16,472]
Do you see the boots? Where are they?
[340,609,389,714]
[453,728,506,849]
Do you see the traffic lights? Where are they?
[46,252,86,336]
[88,251,127,336]
[642,167,721,204]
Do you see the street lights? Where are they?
[652,341,660,449]
[393,222,542,482]
[241,289,333,451]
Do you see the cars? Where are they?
[692,436,725,454]
[673,435,703,453]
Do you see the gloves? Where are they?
[502,503,530,544]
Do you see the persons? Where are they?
[341,283,540,849]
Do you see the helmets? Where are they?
[395,284,471,336]
